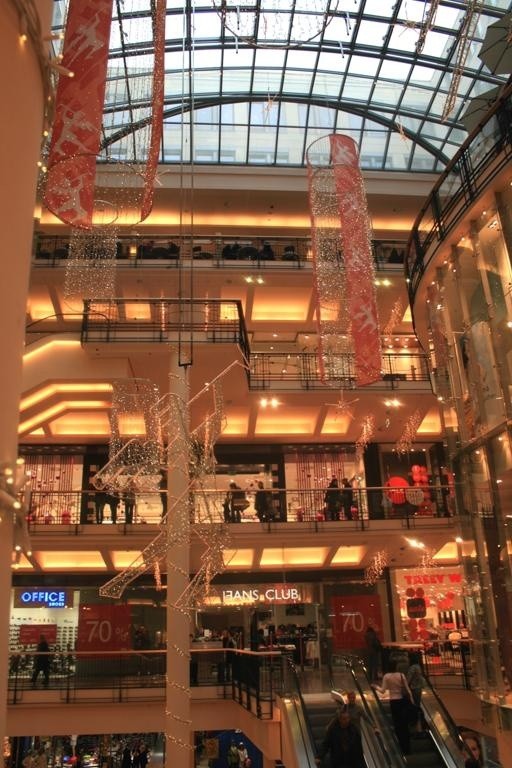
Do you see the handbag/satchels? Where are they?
[400,673,410,705]
[326,703,348,733]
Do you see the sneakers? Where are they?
[411,731,427,739]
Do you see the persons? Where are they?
[322,478,342,520]
[406,651,429,738]
[224,481,278,523]
[370,659,415,754]
[340,478,354,521]
[313,710,364,768]
[29,633,53,684]
[138,237,274,261]
[92,468,168,525]
[22,735,152,767]
[223,630,239,682]
[455,729,480,768]
[340,691,382,737]
[227,739,253,768]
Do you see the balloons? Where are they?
[25,464,76,525]
[411,462,432,517]
[406,586,458,640]
